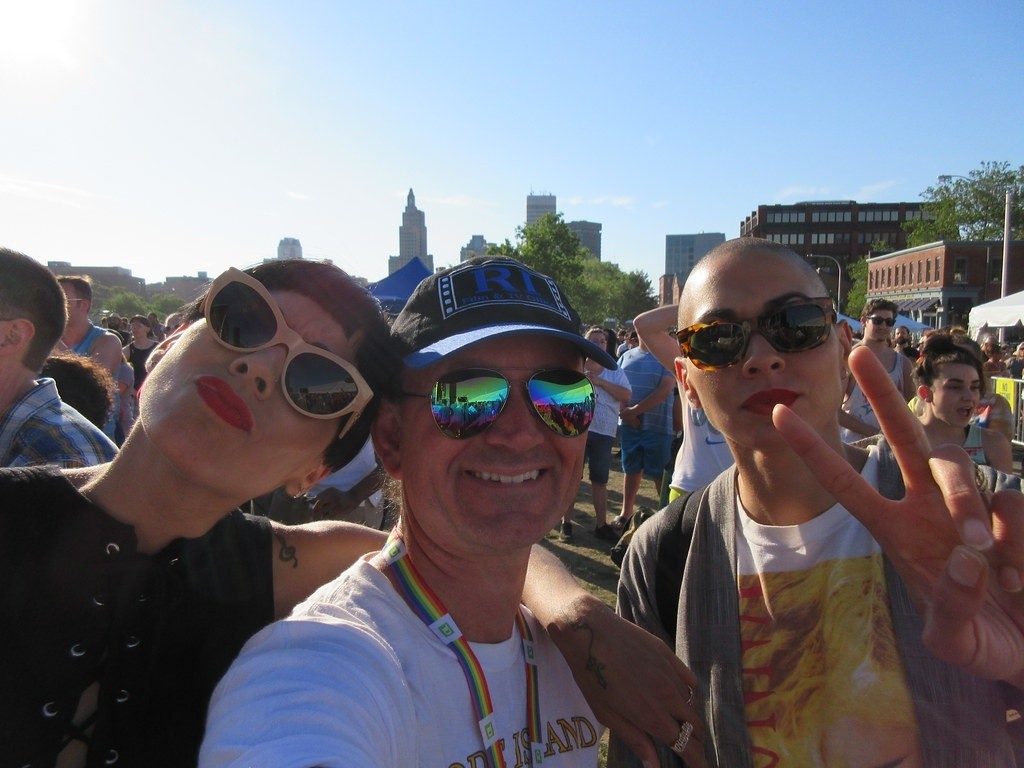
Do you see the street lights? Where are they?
[937,173,1012,348]
[806,253,841,314]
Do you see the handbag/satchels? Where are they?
[610,506,657,570]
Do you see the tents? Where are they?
[967,290,1024,341]
[893,314,933,332]
[364,256,436,315]
[837,313,864,332]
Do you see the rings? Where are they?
[686,684,697,706]
[670,721,694,752]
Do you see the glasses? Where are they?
[402,365,599,439]
[197,267,375,440]
[865,316,896,326]
[676,295,835,370]
[592,339,607,346]
[1020,348,1024,351]
[164,325,180,331]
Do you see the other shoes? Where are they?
[558,519,574,543]
[594,522,621,545]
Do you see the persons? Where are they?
[0,237,1024,768]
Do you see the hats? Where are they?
[107,313,121,322]
[390,255,621,372]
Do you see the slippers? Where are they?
[611,515,627,531]
